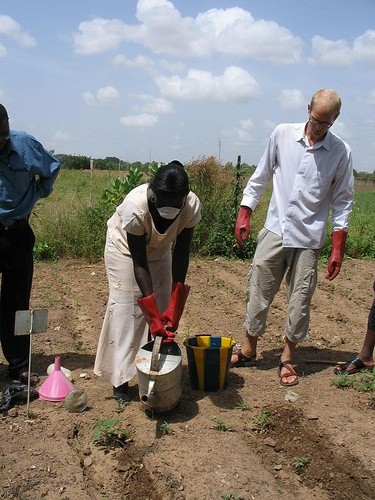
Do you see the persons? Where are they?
[92,160,201,404]
[0,103,62,387]
[333,281,375,375]
[229,87,355,387]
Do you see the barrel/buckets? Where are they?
[183,335,236,392]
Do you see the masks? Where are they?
[155,203,184,219]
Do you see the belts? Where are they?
[0,221,28,229]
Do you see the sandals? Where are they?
[230,350,257,367]
[278,355,298,386]
[20,371,39,385]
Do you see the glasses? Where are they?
[0,129,10,142]
[309,109,335,128]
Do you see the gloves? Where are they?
[325,231,347,281]
[161,282,190,332]
[235,206,252,246]
[137,292,176,343]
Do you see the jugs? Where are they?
[134,325,184,412]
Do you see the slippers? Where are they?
[333,357,374,374]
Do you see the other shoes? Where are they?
[113,382,130,402]
